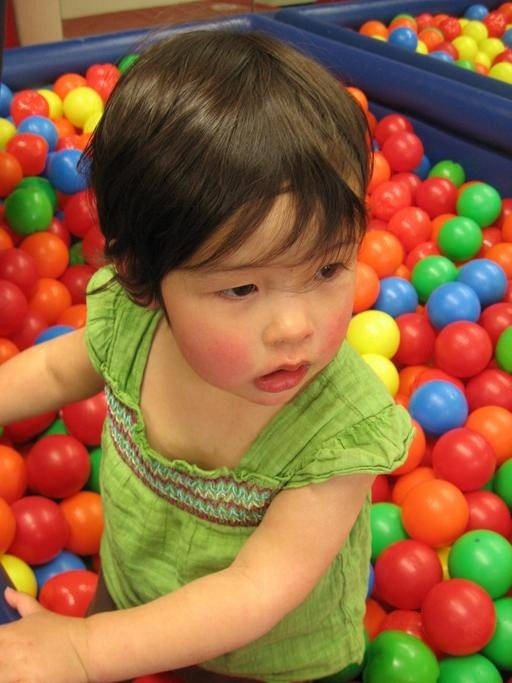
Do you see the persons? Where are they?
[1,25,419,683]
